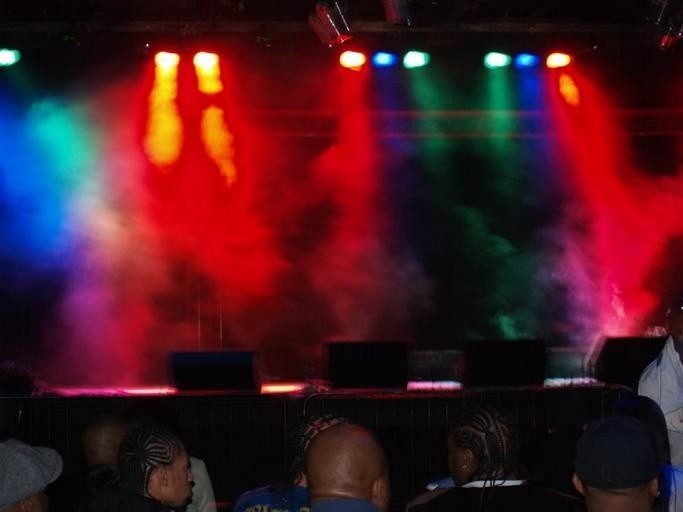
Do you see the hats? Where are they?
[0,437,65,509]
[569,392,672,489]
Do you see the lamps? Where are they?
[315,0,352,46]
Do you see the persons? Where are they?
[231,305,681,511]
[2,417,217,512]
